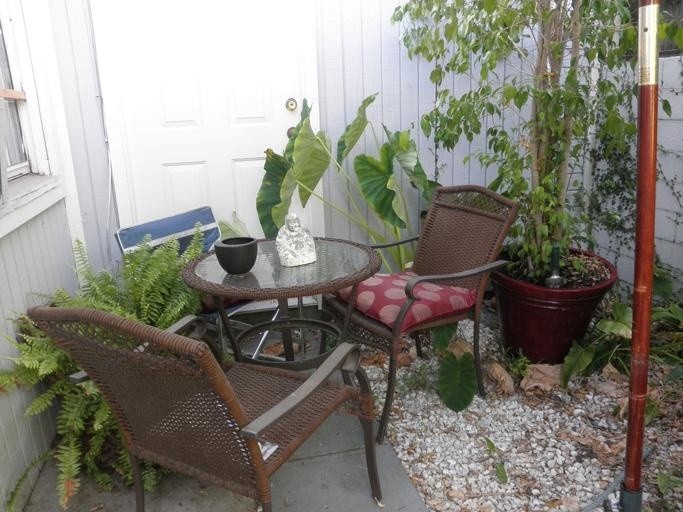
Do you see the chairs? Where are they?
[27,306,381,512]
[320,185,517,444]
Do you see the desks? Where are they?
[182,237,382,389]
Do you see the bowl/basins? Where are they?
[213,235,256,272]
